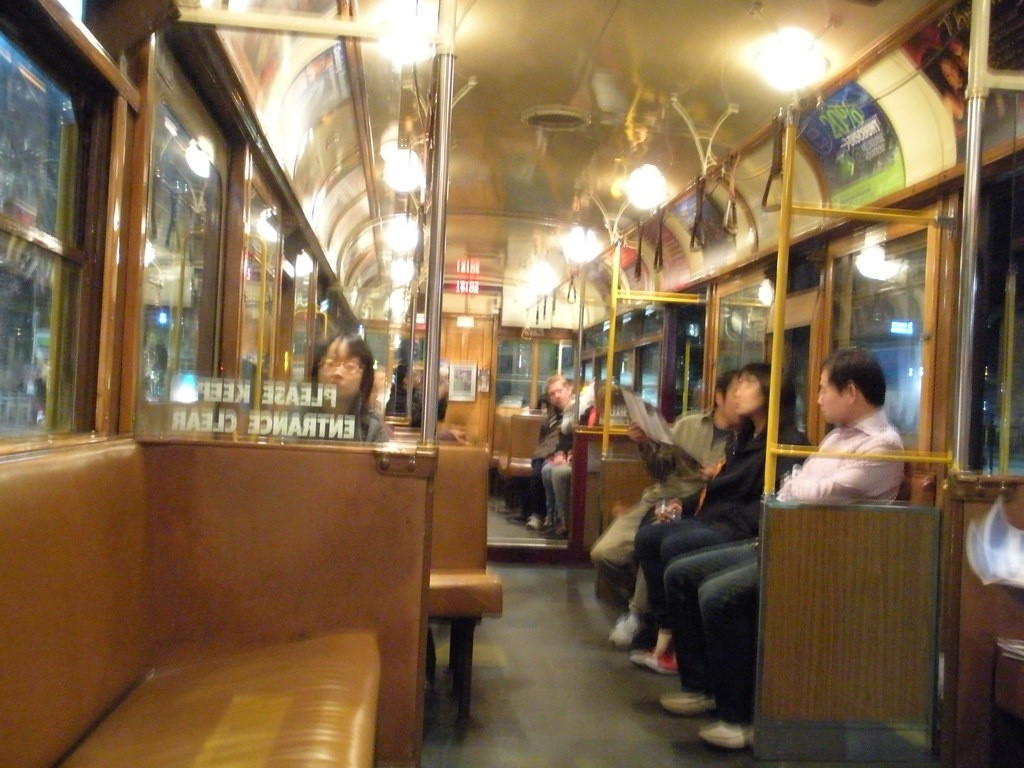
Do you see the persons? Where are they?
[295,332,391,445]
[383,362,451,429]
[588,368,745,649]
[627,364,811,678]
[519,370,622,538]
[653,349,906,748]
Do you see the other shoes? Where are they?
[540,524,560,535]
[645,657,679,675]
[609,619,640,649]
[630,654,657,665]
[545,526,568,539]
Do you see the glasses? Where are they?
[318,357,365,375]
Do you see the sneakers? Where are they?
[527,517,541,530]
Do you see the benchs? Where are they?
[0,404,1024,768]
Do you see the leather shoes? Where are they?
[698,724,754,748]
[660,695,716,715]
